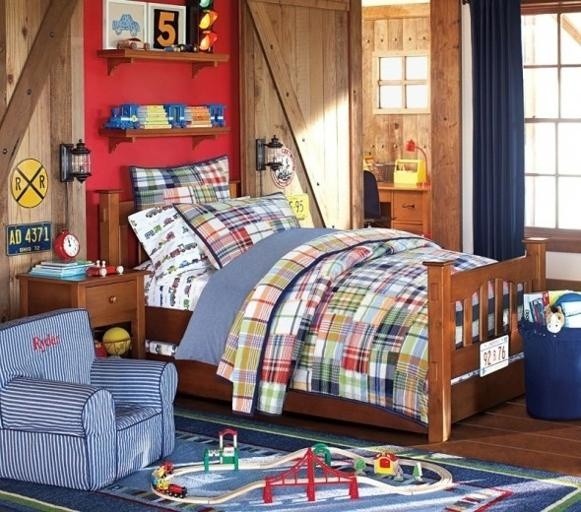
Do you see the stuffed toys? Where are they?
[545,305,565,334]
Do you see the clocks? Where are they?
[53,226,82,265]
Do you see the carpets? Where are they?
[0,401,581,512]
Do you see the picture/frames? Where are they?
[102,0,148,53]
[146,2,189,53]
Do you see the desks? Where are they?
[376,181,434,242]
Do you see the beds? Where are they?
[91,172,554,450]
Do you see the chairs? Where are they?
[363,169,399,232]
[1,303,183,492]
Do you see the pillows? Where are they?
[124,195,253,276]
[171,190,304,271]
[127,154,232,216]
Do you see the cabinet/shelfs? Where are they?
[93,46,234,151]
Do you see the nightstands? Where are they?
[16,260,156,360]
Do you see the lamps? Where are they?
[405,139,433,189]
[56,137,94,185]
[254,135,286,172]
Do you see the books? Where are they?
[138,104,172,129]
[524,289,574,326]
[29,259,96,277]
[184,106,213,128]
